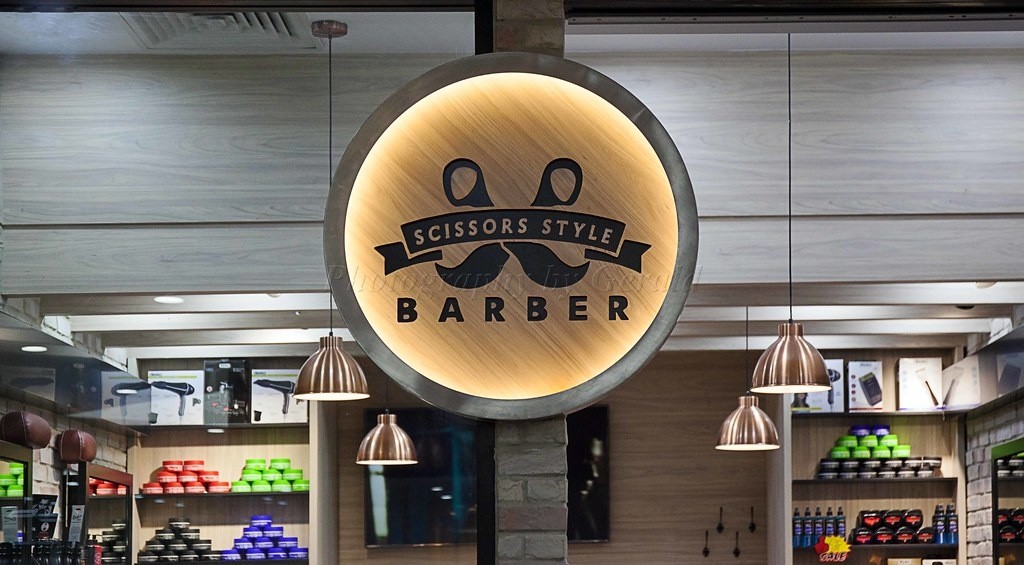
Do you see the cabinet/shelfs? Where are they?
[991,438,1024,565]
[767,410,967,564]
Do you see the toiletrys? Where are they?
[0,539,102,565]
[850,509,934,544]
[230,458,309,492]
[815,423,942,479]
[791,506,848,546]
[1,505,18,543]
[0,463,24,497]
[31,494,59,539]
[89,477,127,495]
[996,507,1024,542]
[67,504,85,540]
[136,517,221,561]
[142,458,229,495]
[221,515,308,560]
[932,503,957,544]
[100,518,127,562]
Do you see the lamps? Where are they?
[0,381,344,564]
[715,305,781,451]
[355,381,419,465]
[292,21,370,402]
[750,33,831,394]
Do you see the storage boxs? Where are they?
[791,358,845,413]
[146,370,205,425]
[251,368,308,423]
[847,360,884,411]
[896,358,942,410]
[204,360,252,423]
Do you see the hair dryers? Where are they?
[150,381,194,416]
[816,367,841,404]
[111,381,149,417]
[252,379,297,414]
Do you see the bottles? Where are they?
[814,506,825,547]
[946,502,958,544]
[931,503,945,543]
[792,506,802,546]
[836,506,846,540]
[825,506,836,537]
[803,506,813,547]
[17,530,23,543]
